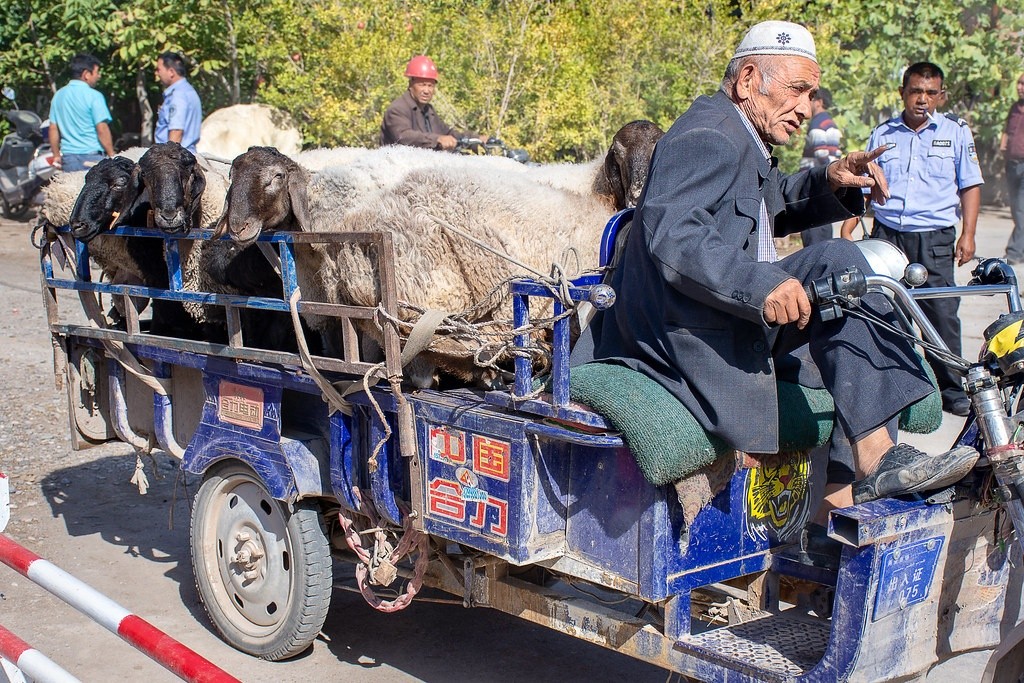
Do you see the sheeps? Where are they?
[41,120,667,391]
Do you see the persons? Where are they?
[48,54,115,173]
[798,88,840,247]
[154,53,202,155]
[989,73,1024,265]
[379,55,498,153]
[840,62,986,418]
[606,21,980,506]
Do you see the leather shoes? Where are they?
[852,442,980,504]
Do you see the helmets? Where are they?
[404,55,438,82]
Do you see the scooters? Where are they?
[0,86,66,218]
[437,134,544,169]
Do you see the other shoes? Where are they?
[90,260,99,268]
[1003,256,1017,265]
[942,396,973,415]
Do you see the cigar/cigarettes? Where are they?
[923,108,936,122]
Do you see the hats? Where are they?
[732,20,818,64]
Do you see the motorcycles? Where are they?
[22,226,1023,683]
